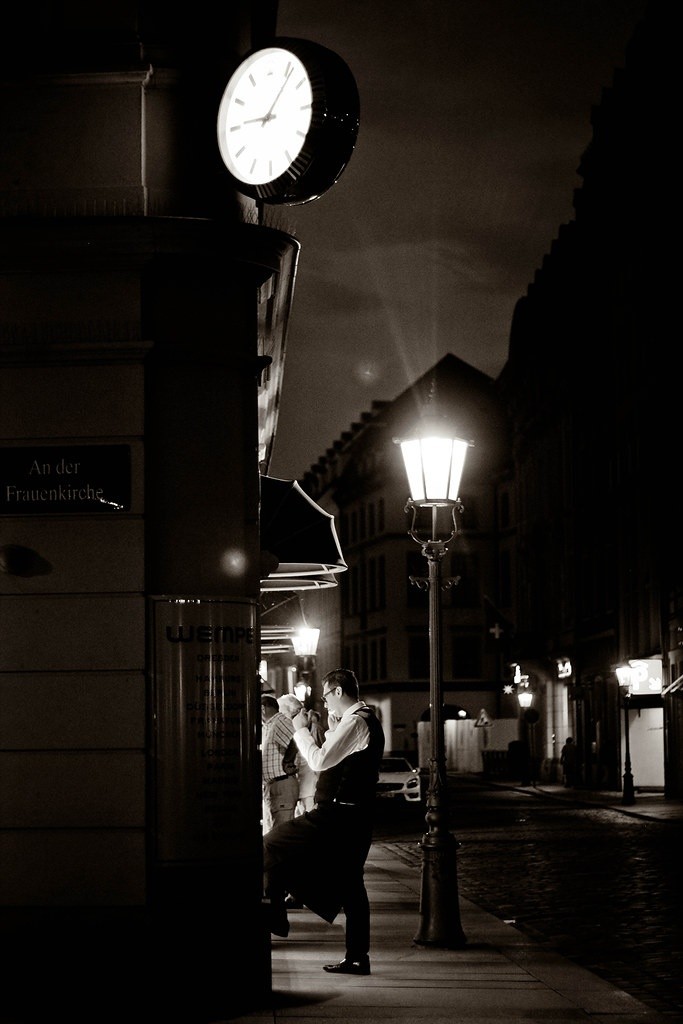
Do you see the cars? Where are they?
[377,758,421,813]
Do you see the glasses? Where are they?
[321,688,336,702]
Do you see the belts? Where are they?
[272,775,289,781]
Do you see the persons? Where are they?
[261,668,385,975]
[560,737,576,787]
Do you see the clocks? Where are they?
[216,37,359,208]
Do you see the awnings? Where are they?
[259,475,348,591]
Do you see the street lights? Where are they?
[290,625,321,711]
[616,660,636,807]
[391,378,474,948]
[518,690,533,786]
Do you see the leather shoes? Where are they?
[272,919,290,938]
[323,955,370,975]
